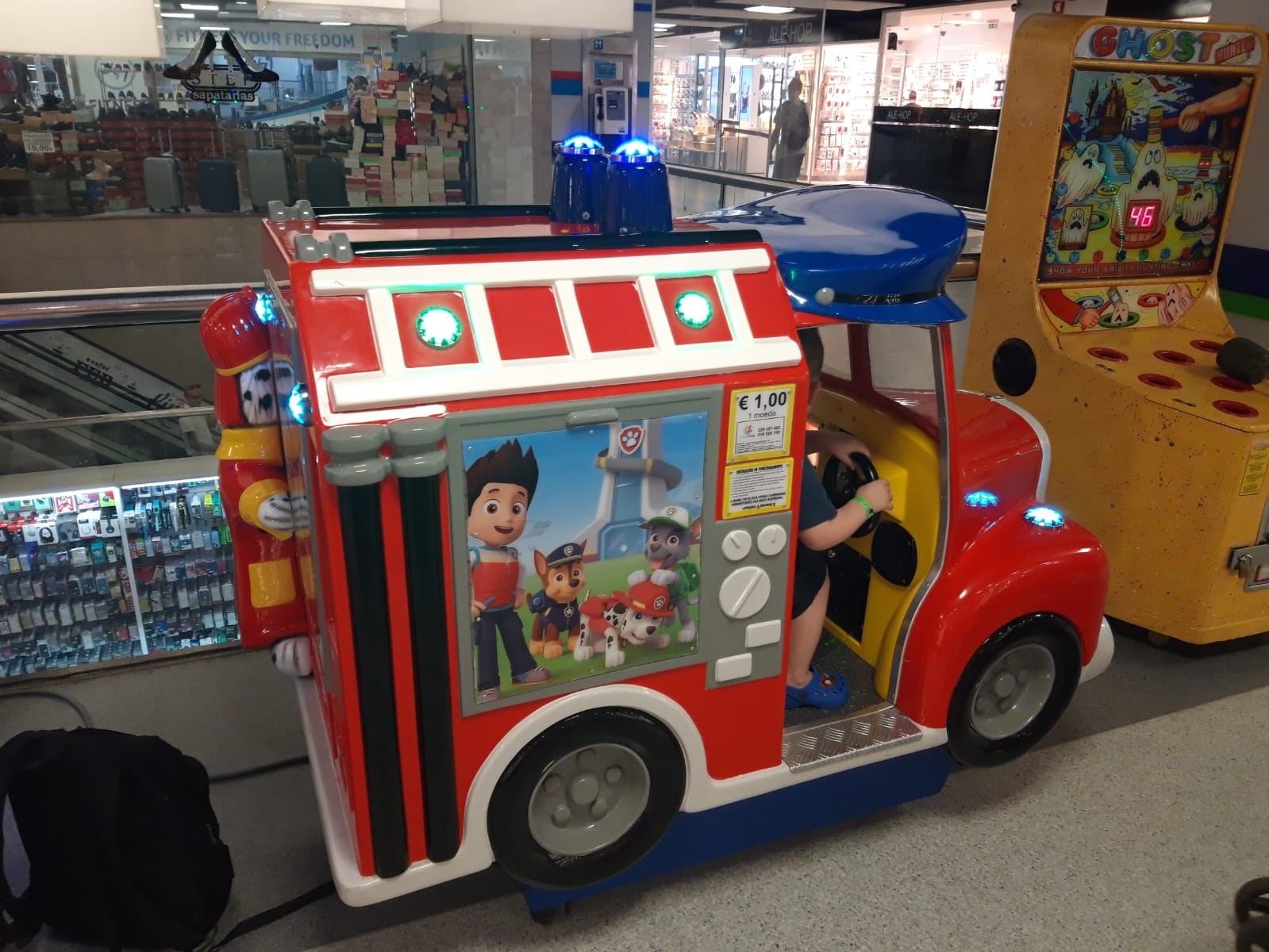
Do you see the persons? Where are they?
[785,327,894,708]
[349,76,383,134]
[769,73,810,182]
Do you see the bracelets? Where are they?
[852,495,874,518]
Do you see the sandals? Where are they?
[786,666,851,709]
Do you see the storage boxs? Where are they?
[321,69,469,207]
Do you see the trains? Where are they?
[200,136,1114,920]
